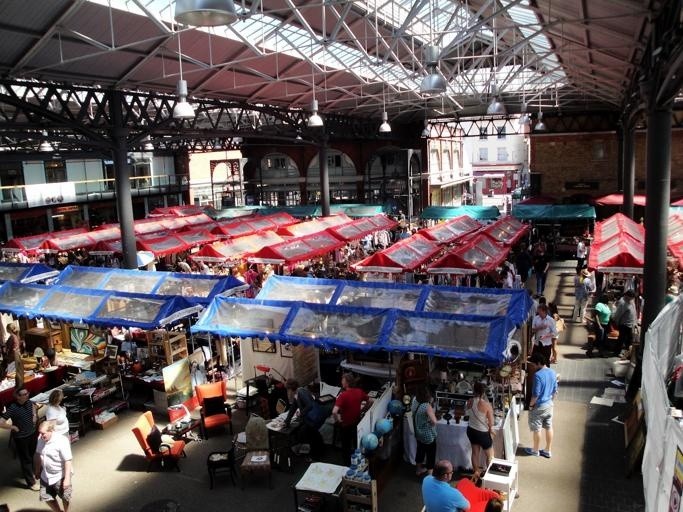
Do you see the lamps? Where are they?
[518,41,534,127]
[376,3,397,133]
[535,74,549,133]
[168,19,197,119]
[173,3,238,27]
[420,0,449,95]
[483,2,506,115]
[302,8,327,129]
[419,86,433,140]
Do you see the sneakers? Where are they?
[30,484,41,491]
[539,449,551,458]
[550,359,558,364]
[524,448,540,458]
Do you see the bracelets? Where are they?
[31,414,37,417]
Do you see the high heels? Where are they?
[473,471,479,484]
[586,352,596,358]
[598,350,609,358]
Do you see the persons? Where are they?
[489,226,595,322]
[531,303,557,367]
[524,353,559,458]
[332,373,375,466]
[1,388,41,490]
[467,381,496,481]
[34,421,73,512]
[1,323,20,370]
[283,378,326,441]
[548,302,564,364]
[190,360,205,390]
[422,460,470,512]
[46,390,70,439]
[612,289,638,357]
[586,293,613,358]
[290,209,416,280]
[411,384,438,476]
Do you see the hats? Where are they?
[581,269,592,277]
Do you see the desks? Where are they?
[162,417,205,440]
[244,375,281,418]
[432,407,503,470]
[207,434,238,487]
[266,408,301,470]
[0,365,67,414]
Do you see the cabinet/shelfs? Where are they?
[342,457,382,512]
[147,332,187,364]
[79,375,128,430]
[292,461,349,512]
[481,456,523,511]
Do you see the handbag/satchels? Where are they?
[469,398,497,441]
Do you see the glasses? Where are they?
[444,471,454,475]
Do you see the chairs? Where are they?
[195,381,234,437]
[240,413,277,491]
[132,412,189,473]
